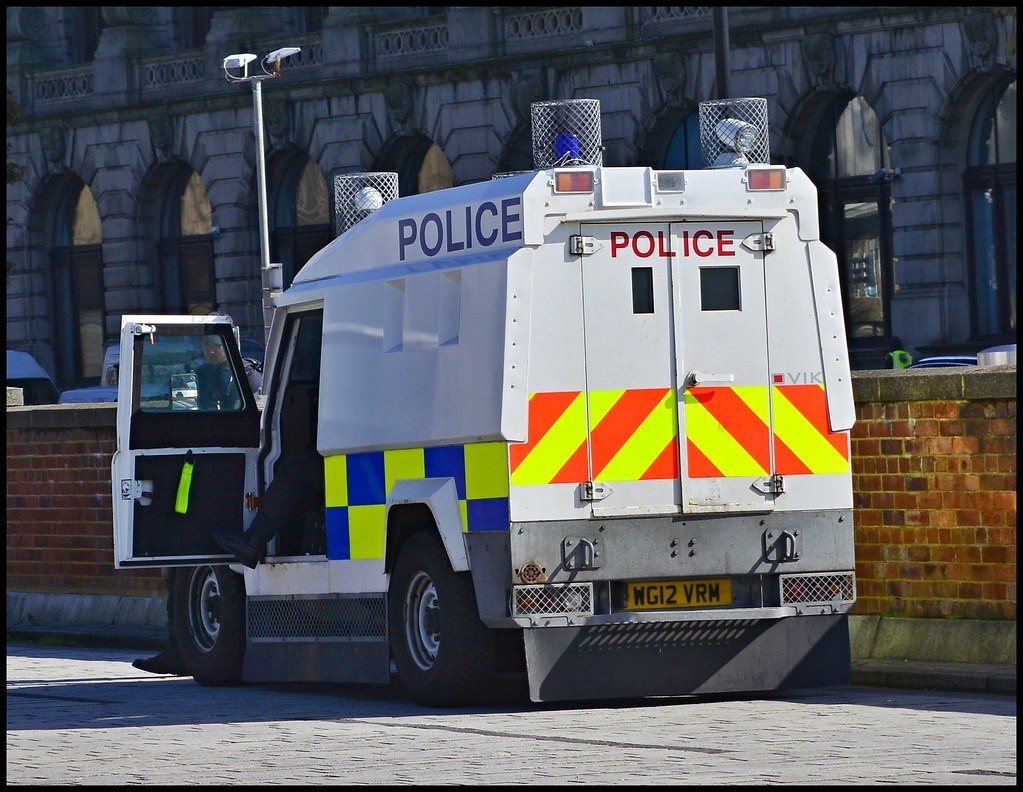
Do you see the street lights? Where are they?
[222,46,301,350]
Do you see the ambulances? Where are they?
[111,99,858,705]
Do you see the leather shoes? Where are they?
[211,527,260,569]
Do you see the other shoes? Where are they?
[132,652,184,676]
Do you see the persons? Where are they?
[884,336,912,370]
[133,310,261,678]
